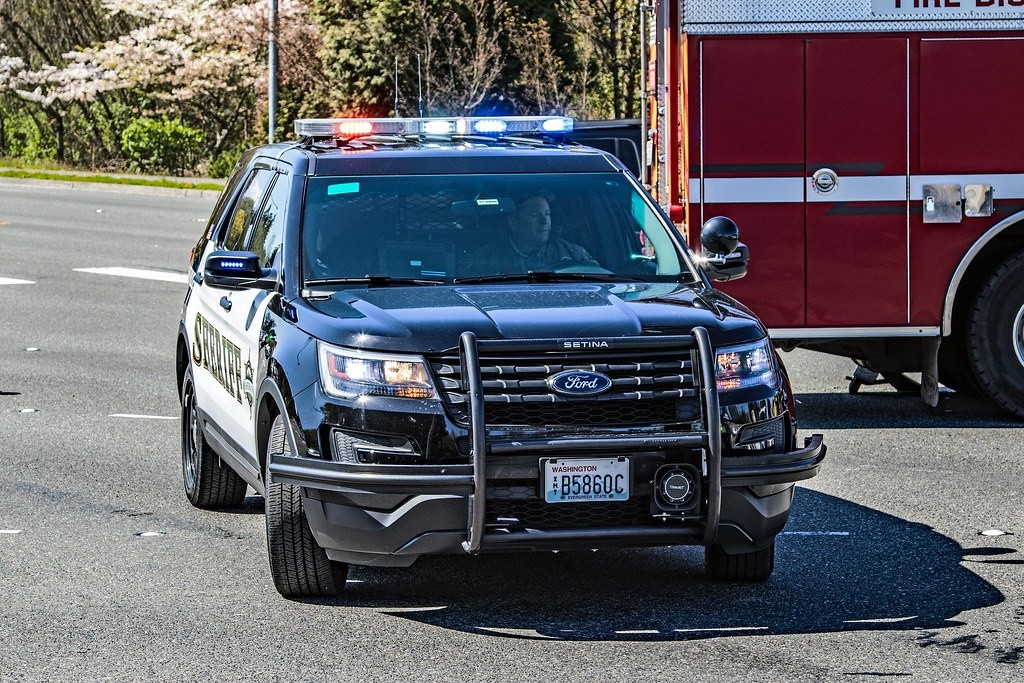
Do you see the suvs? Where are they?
[175,113,828,604]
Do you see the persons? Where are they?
[473,191,599,277]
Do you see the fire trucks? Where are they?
[632,1,1024,424]
